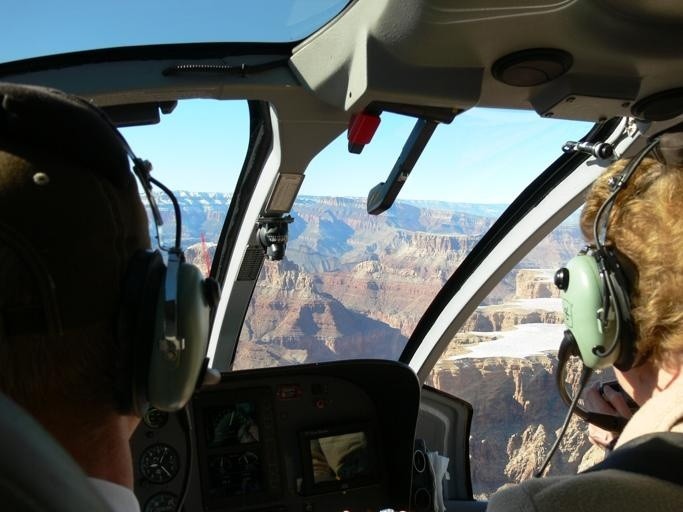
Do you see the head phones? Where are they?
[555,87,683,373]
[0,84,221,413]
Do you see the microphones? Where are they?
[555,329,628,431]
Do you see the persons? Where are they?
[0,80,221,512]
[582,380,633,461]
[483,89,683,512]
